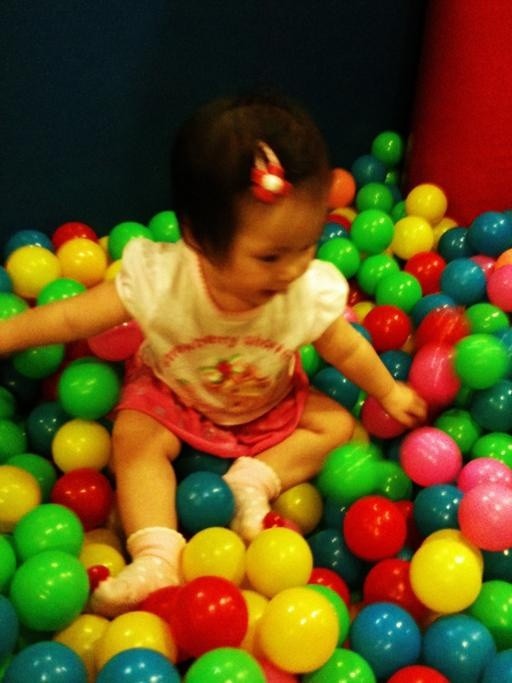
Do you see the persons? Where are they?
[0,102,427,611]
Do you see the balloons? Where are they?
[376,462,408,498]
[350,387,362,419]
[237,590,269,655]
[300,342,320,374]
[313,492,512,678]
[458,340,504,390]
[153,210,179,244]
[254,654,298,682]
[101,609,177,663]
[320,131,512,310]
[14,343,62,376]
[173,575,252,656]
[458,457,510,489]
[467,303,508,334]
[400,425,461,487]
[274,485,321,531]
[182,647,264,682]
[469,380,512,432]
[364,393,403,439]
[182,526,246,588]
[259,588,338,672]
[175,471,236,531]
[1,388,124,681]
[416,308,468,347]
[87,320,140,361]
[98,647,179,682]
[0,221,152,317]
[469,432,512,470]
[315,364,357,407]
[317,442,373,497]
[57,356,119,418]
[240,526,314,595]
[381,349,411,379]
[435,408,477,455]
[362,305,411,351]
[413,343,461,403]
